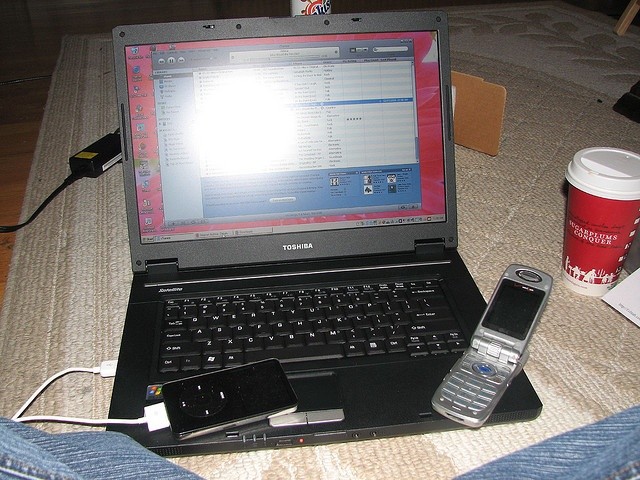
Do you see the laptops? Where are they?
[110,10,543,457]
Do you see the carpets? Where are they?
[0,3,639,479]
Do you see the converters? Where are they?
[66,135,119,176]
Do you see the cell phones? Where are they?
[428,260,553,423]
[160,357,298,441]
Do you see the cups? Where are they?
[560,145,640,299]
